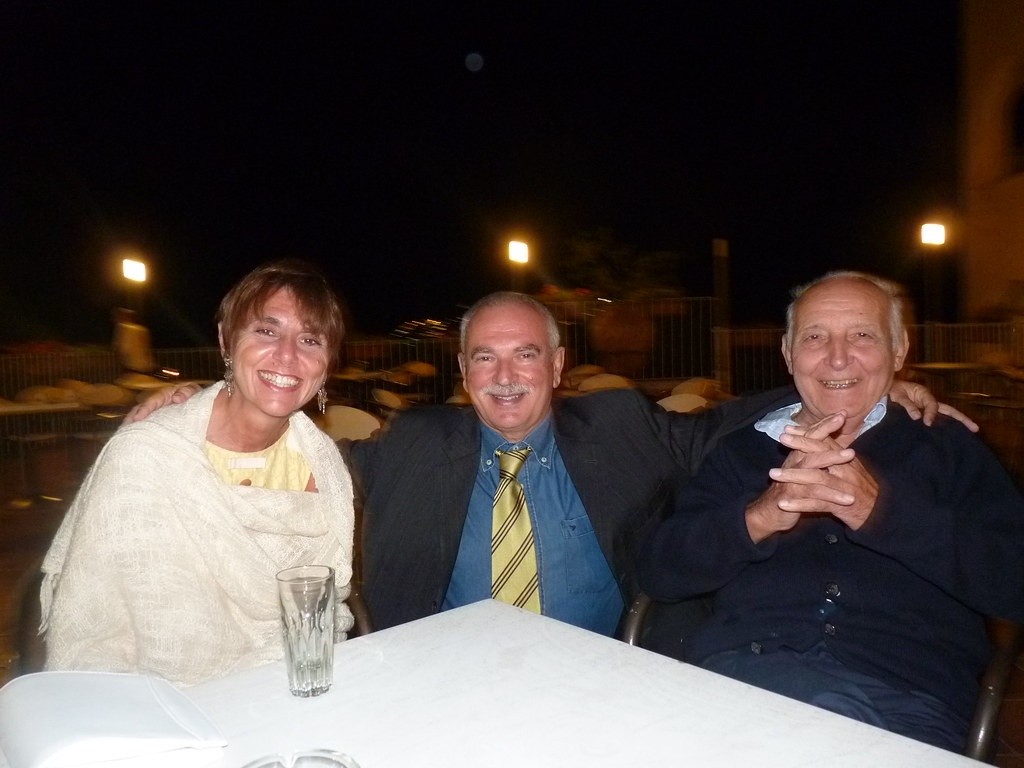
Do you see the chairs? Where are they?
[655,394,709,412]
[578,373,628,393]
[308,405,382,507]
[672,378,709,396]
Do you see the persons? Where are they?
[33,258,356,691]
[631,270,1024,755]
[118,291,980,640]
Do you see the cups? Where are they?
[276,565,335,697]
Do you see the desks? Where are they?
[0,597,999,768]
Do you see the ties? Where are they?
[492,445,541,617]
